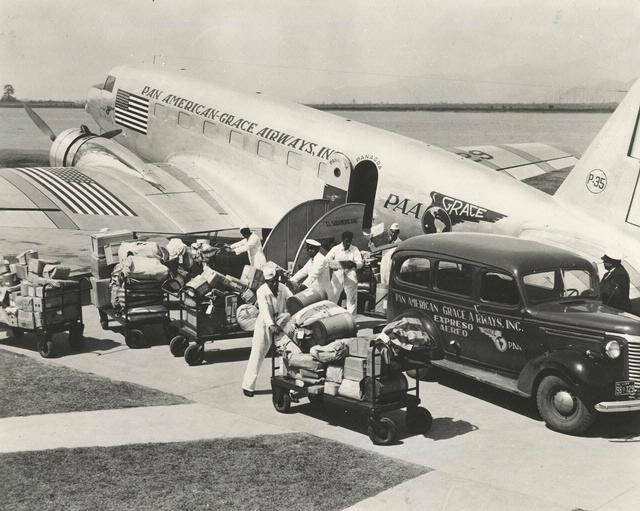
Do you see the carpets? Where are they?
[1,430,436,510]
[1,349,196,417]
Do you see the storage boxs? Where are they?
[322,336,374,399]
[179,260,265,321]
[370,222,390,247]
[0,249,94,330]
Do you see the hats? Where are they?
[240,225,248,229]
[600,250,622,264]
[261,264,276,280]
[390,223,400,230]
[305,238,321,247]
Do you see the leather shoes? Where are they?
[289,393,298,402]
[243,390,253,397]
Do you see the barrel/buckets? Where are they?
[311,311,358,346]
[285,285,329,316]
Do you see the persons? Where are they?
[325,230,364,316]
[224,224,267,268]
[242,265,293,398]
[367,222,403,285]
[287,238,333,301]
[600,250,630,312]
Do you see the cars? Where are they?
[385,232,640,434]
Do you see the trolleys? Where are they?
[253,334,431,446]
[98,243,308,366]
[0,277,92,358]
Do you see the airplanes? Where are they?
[0,65,640,300]
